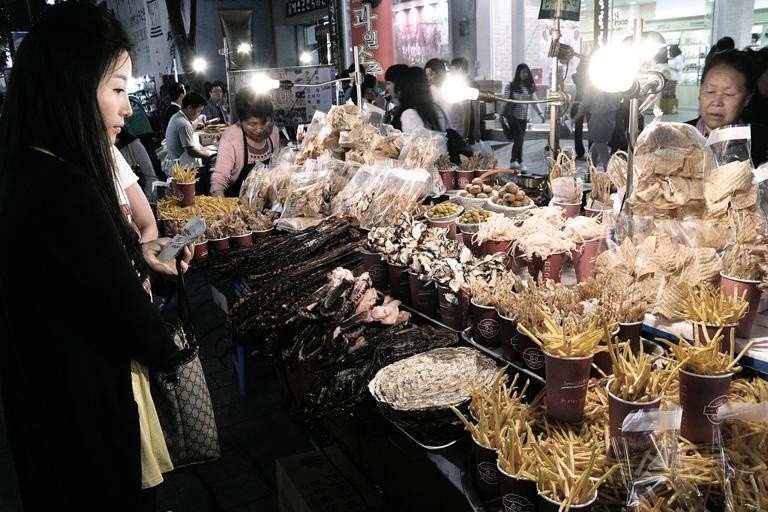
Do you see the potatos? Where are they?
[460,177,530,207]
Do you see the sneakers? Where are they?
[510,160,529,173]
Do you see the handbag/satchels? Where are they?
[444,126,472,164]
[503,84,514,119]
[149,249,221,471]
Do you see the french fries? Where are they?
[449,282,768,512]
[157,162,238,224]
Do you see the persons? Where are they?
[337,56,483,167]
[570,33,767,183]
[499,63,546,175]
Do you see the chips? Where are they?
[594,123,768,321]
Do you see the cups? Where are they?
[351,169,767,512]
[152,177,275,265]
